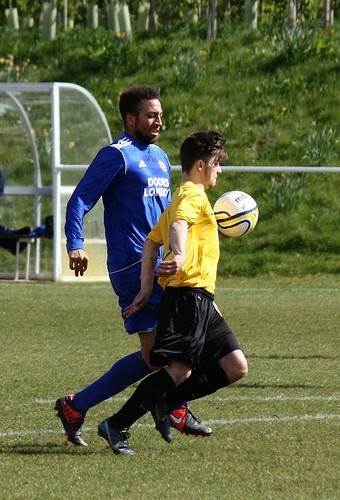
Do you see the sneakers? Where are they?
[54,394,89,447]
[150,391,171,443]
[98,417,137,456]
[170,401,212,438]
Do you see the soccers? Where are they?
[211,189,262,239]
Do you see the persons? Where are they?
[54,87,213,447]
[98,131,249,457]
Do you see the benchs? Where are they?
[13,235,53,284]
